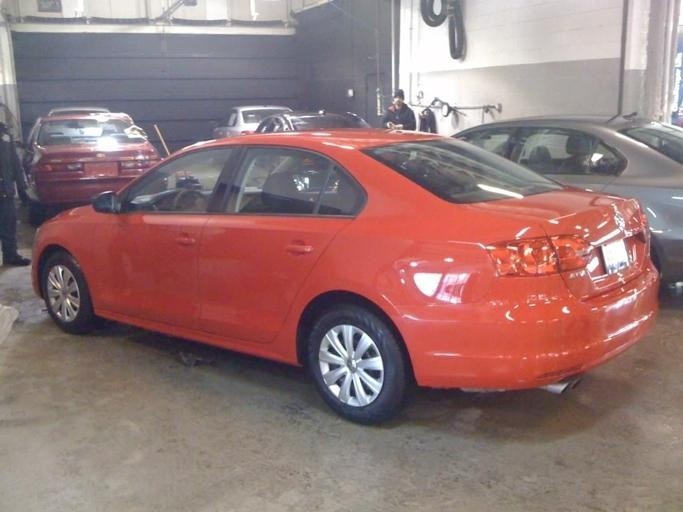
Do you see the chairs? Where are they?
[244,169,357,214]
[525,134,599,177]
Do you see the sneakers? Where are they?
[3,254,31,266]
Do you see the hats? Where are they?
[394,89,403,98]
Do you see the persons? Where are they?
[0,122,32,266]
[383,89,416,130]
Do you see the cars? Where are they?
[256,111,373,131]
[212,106,294,139]
[29,128,663,423]
[21,112,171,209]
[45,107,111,117]
[274,118,683,282]
